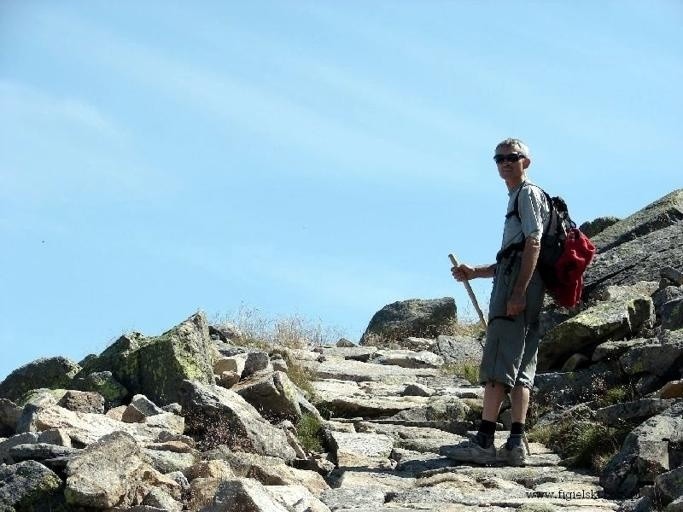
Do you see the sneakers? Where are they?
[440,435,527,466]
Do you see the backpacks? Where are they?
[515,184,594,307]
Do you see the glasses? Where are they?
[494,153,525,163]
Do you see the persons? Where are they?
[435,136,557,470]
